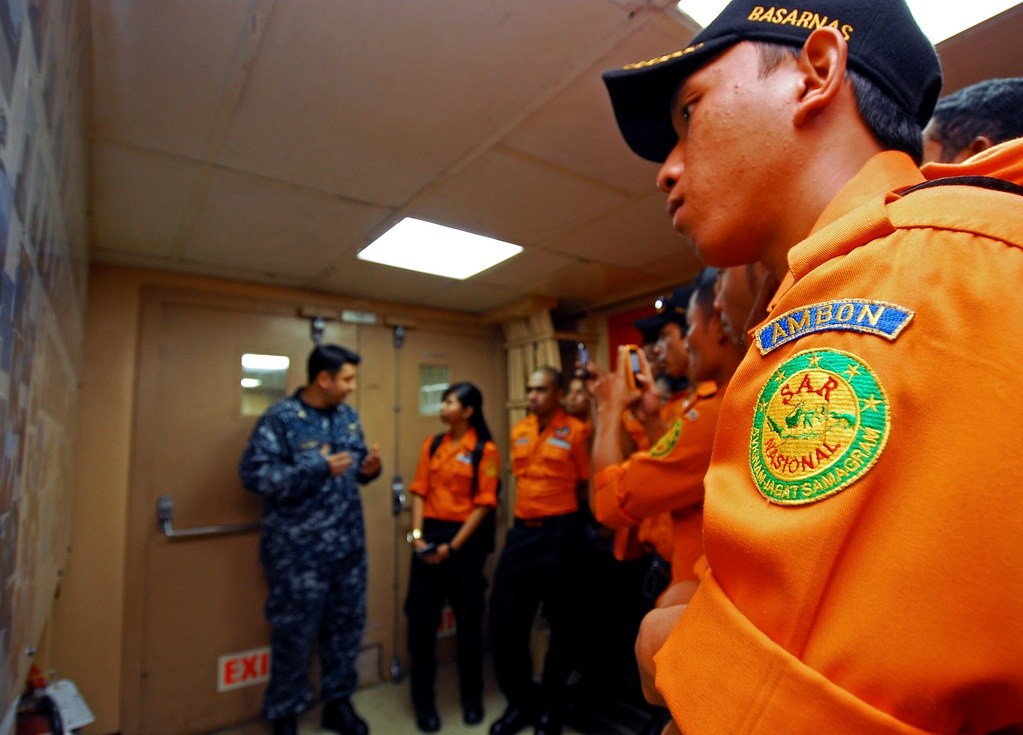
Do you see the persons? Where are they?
[237,342,384,735]
[490,79,1023,735]
[403,380,501,732]
[603,1,1022,735]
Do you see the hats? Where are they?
[630,288,694,336]
[598,0,942,165]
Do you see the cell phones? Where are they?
[578,343,590,381]
[626,345,644,390]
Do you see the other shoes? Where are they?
[460,685,486,725]
[532,709,564,735]
[264,714,301,735]
[411,692,442,732]
[318,700,369,735]
[490,703,539,735]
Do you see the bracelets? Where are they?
[408,528,420,540]
